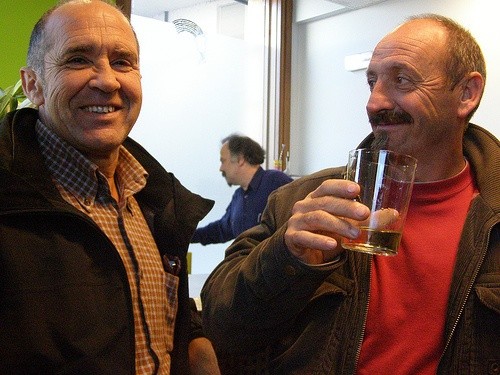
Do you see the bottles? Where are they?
[278,144,289,177]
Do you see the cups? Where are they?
[340,149,418,257]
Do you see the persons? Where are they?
[200,14,499,375]
[1,0,221,375]
[189,134,296,246]
